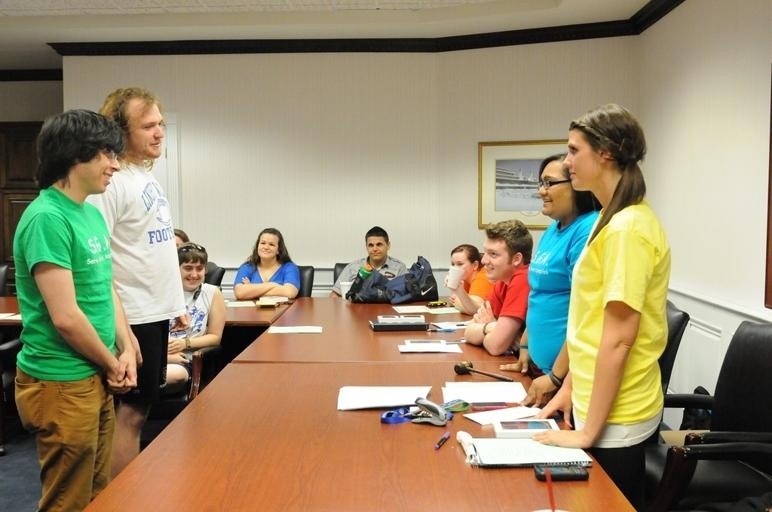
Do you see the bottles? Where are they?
[358,265,372,281]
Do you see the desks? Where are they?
[0,296,295,338]
[232,296,519,363]
[81,361,639,511]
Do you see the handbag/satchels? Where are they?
[679,386,711,430]
[345,256,438,303]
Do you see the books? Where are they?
[476,437,593,467]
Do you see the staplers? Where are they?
[411,397,448,427]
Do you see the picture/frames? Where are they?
[151,113,181,232]
[0,195,40,267]
[477,139,569,229]
[0,120,45,193]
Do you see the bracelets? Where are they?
[519,345,528,349]
[483,322,487,335]
[549,371,563,388]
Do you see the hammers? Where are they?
[455,361,516,383]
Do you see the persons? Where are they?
[162,229,227,398]
[330,226,407,297]
[14,109,137,510]
[502,152,604,408]
[530,106,671,511]
[463,219,533,357]
[444,244,493,315]
[86,88,189,483]
[233,228,301,299]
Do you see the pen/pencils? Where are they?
[435,433,450,450]
[428,328,456,332]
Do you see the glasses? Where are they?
[178,246,205,254]
[538,180,568,190]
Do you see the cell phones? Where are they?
[534,464,589,481]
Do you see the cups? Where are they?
[446,267,465,289]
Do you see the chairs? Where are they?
[202,262,225,285]
[0,338,22,455]
[649,300,691,442]
[296,266,314,298]
[644,321,771,511]
[334,263,348,280]
[2,265,9,292]
[146,346,225,419]
